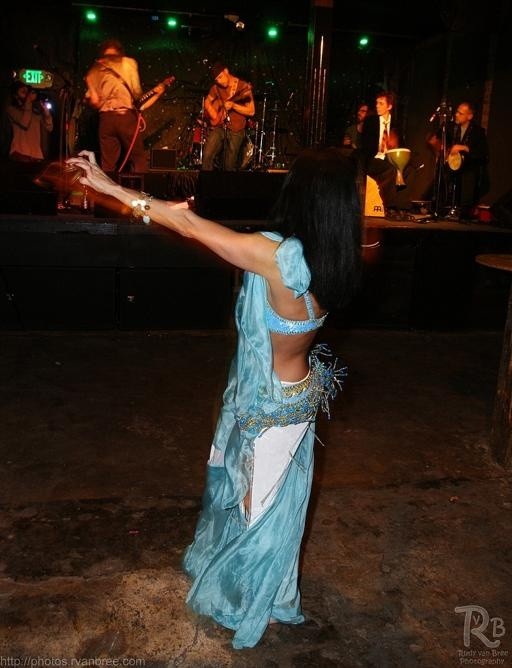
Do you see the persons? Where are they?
[434,99,491,218]
[342,104,370,148]
[357,90,406,198]
[84,37,165,174]
[200,59,256,172]
[61,150,363,650]
[4,78,54,164]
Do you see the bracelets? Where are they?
[132,190,153,226]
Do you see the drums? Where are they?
[386,148,412,186]
[193,129,207,145]
[213,134,253,170]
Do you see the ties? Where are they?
[381,122,388,153]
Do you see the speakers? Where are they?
[150,149,177,170]
[194,172,289,220]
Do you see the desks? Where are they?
[474,253,512,470]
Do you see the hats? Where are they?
[211,63,225,79]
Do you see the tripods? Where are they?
[197,88,284,173]
[417,123,471,226]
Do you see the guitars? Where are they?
[133,77,175,110]
[205,83,251,126]
